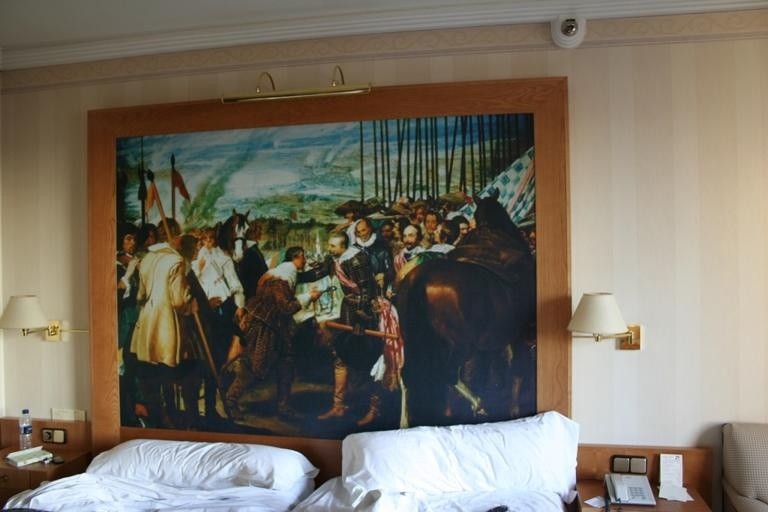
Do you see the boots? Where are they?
[357,395,379,425]
[224,377,244,420]
[278,383,296,417]
[318,365,348,420]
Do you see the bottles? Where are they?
[19,409,33,450]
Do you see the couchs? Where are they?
[720,422,768,511]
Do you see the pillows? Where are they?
[340,410,580,509]
[86,438,321,491]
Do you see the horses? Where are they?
[219,207,268,299]
[397,187,538,429]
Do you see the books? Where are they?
[6,445,54,468]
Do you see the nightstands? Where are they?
[0,446,85,511]
[576,478,711,511]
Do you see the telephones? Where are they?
[604,473,656,506]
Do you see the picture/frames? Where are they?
[85,76,574,457]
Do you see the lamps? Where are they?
[0,295,61,341]
[567,292,641,350]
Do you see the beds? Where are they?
[4,472,565,511]
